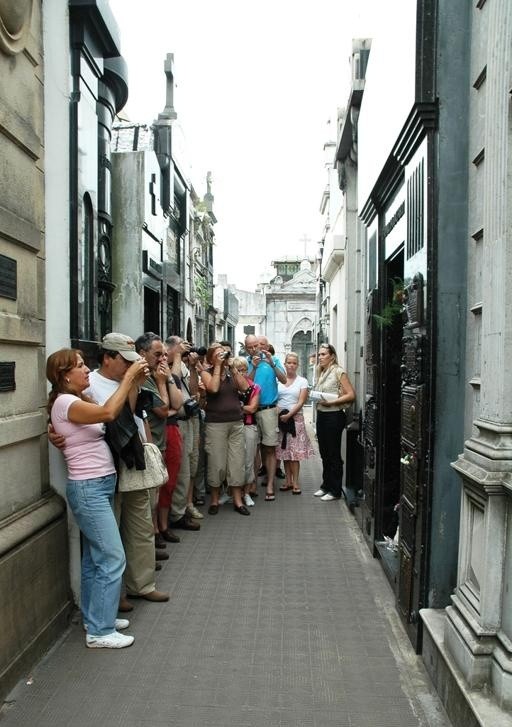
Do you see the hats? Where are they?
[101,332,142,362]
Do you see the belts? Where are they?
[258,405,277,412]
[176,415,190,420]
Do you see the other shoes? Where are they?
[292,484,301,495]
[169,515,200,531]
[186,503,203,518]
[275,468,285,479]
[265,488,274,500]
[154,533,167,549]
[279,484,293,492]
[217,493,234,505]
[155,563,161,571]
[243,493,254,506]
[248,491,258,498]
[126,590,170,602]
[119,598,133,613]
[160,529,180,543]
[320,494,338,500]
[194,496,205,506]
[314,490,324,496]
[257,466,267,476]
[208,502,219,515]
[261,477,268,486]
[234,504,250,515]
[155,551,169,561]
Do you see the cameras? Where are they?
[260,353,266,359]
[184,397,200,416]
[182,345,207,356]
[223,351,233,358]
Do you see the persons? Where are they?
[47,349,150,648]
[137,332,316,548]
[46,333,170,612]
[311,344,356,501]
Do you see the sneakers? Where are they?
[84,619,130,630]
[86,631,134,649]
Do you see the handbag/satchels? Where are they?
[118,443,169,493]
[338,383,352,409]
